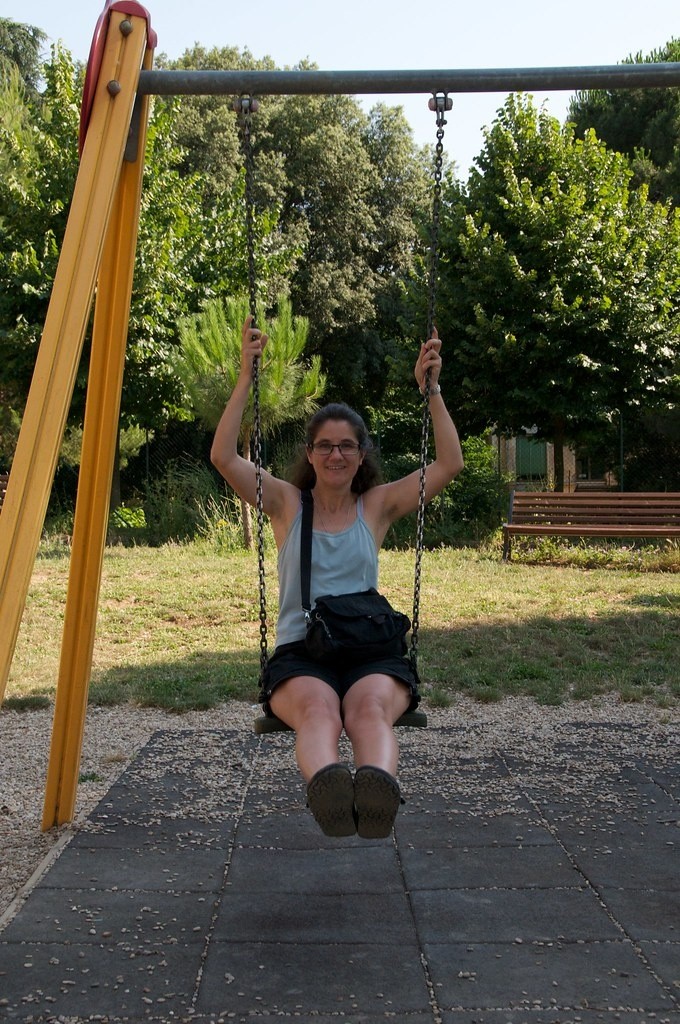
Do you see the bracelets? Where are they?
[419,384,440,396]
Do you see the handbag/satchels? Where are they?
[304,587,412,674]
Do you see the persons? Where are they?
[211,314,464,839]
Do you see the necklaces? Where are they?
[311,489,354,534]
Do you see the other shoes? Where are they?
[354,766,406,839]
[305,763,357,837]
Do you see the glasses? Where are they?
[308,442,361,455]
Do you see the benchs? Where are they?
[497,490,680,560]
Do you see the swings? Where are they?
[231,91,455,736]
